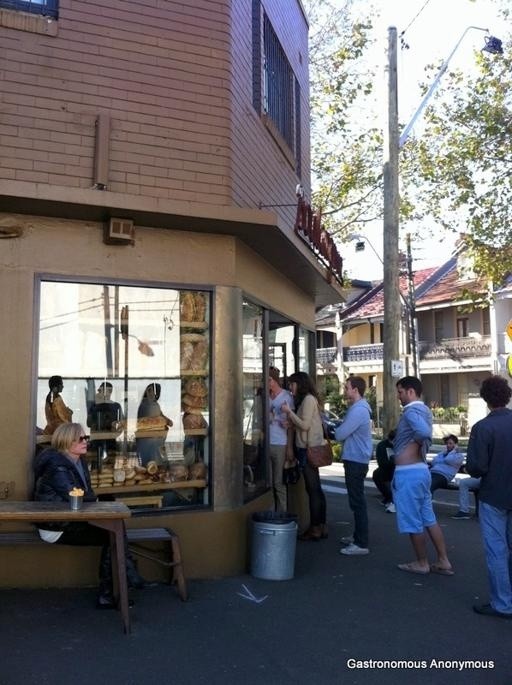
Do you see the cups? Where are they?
[68,490,84,511]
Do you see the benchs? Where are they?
[426,460,479,517]
[0,526,189,602]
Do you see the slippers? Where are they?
[397,562,430,575]
[431,565,455,576]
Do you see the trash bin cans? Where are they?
[248,511,298,581]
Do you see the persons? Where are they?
[466,374,512,618]
[375,428,396,513]
[450,477,482,520]
[182,412,207,460]
[333,375,374,556]
[280,371,328,540]
[86,381,125,451]
[427,434,464,492]
[390,376,455,576]
[268,364,296,514]
[31,422,135,609]
[134,383,173,467]
[44,376,74,435]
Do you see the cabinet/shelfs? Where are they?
[36,314,208,495]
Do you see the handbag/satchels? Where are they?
[307,443,333,467]
[282,465,300,485]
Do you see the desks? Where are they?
[0,500,132,635]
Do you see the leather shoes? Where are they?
[297,526,321,540]
[321,525,328,539]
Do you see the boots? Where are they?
[97,546,135,609]
[126,536,158,590]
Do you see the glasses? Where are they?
[78,436,90,444]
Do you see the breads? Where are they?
[83,290,208,488]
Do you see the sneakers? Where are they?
[451,511,471,520]
[339,536,355,544]
[385,502,396,513]
[340,544,369,555]
[474,604,512,618]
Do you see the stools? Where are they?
[115,496,164,509]
